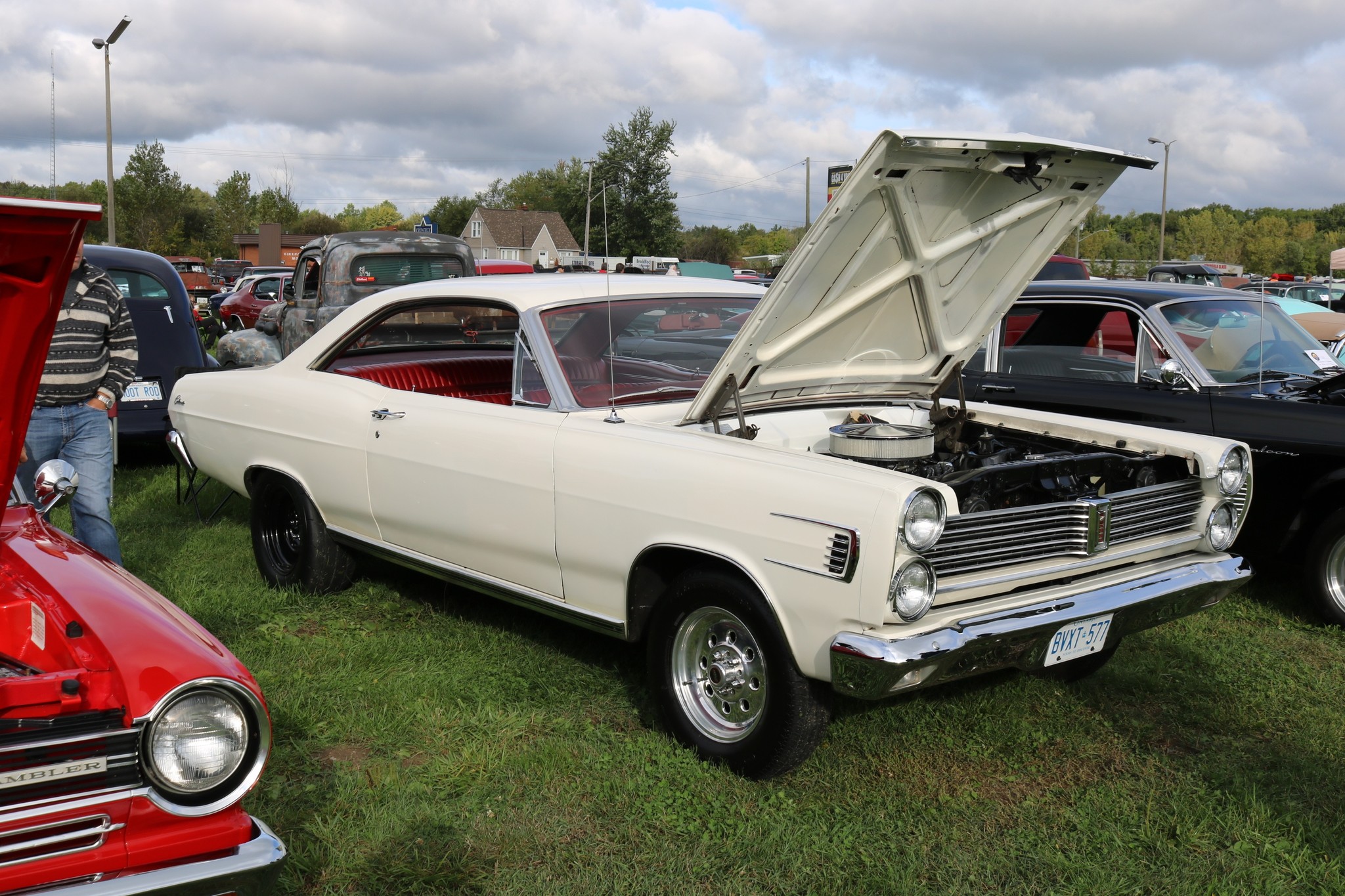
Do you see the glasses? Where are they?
[190,300,195,303]
[561,268,563,270]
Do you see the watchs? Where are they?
[93,394,114,410]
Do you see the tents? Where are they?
[1328,247,1345,312]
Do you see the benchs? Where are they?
[964,349,1065,377]
[1080,369,1161,383]
[460,379,706,410]
[254,295,270,300]
[333,356,609,398]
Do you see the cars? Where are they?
[1002,253,1345,370]
[945,279,1345,629]
[161,229,802,371]
[82,244,223,466]
[0,196,288,896]
[169,125,1258,781]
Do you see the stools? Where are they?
[199,327,208,345]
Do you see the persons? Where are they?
[555,265,566,273]
[764,270,771,288]
[598,263,625,273]
[15,238,138,570]
[665,264,677,275]
[1270,273,1279,281]
[1305,273,1313,284]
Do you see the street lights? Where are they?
[91,13,132,248]
[583,184,618,266]
[1148,136,1177,265]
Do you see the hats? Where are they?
[556,265,565,269]
[1272,273,1279,279]
